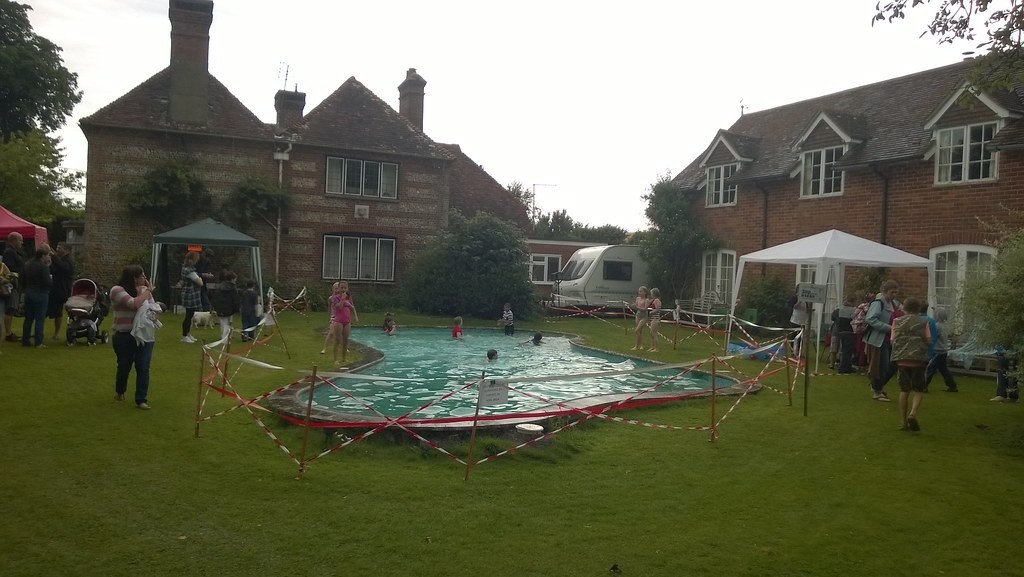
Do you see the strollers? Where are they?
[64,278,110,347]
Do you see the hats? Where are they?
[864,293,875,302]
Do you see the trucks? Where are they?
[550,244,647,318]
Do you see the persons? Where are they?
[320,280,359,366]
[452,316,463,340]
[383,311,396,337]
[215,271,259,345]
[497,303,515,337]
[989,344,1022,403]
[194,249,214,312]
[890,296,934,433]
[487,348,499,360]
[786,282,814,363]
[519,332,546,347]
[108,265,167,410]
[0,232,75,349]
[829,279,959,402]
[179,250,203,343]
[630,286,662,353]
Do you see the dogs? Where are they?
[190,311,218,329]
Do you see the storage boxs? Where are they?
[174,305,186,314]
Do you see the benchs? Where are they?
[675,290,730,330]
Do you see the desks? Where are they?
[167,286,217,314]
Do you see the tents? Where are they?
[0,205,48,255]
[149,216,265,318]
[725,226,936,359]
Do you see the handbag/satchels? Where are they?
[825,323,835,346]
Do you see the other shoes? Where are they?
[945,388,959,392]
[907,414,920,431]
[35,343,48,349]
[988,396,1020,403]
[872,392,893,402]
[113,392,125,404]
[181,334,198,343]
[5,333,20,342]
[22,341,35,347]
[837,370,852,376]
[135,403,152,410]
[897,424,908,430]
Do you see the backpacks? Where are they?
[885,309,905,344]
[848,299,884,334]
[784,294,809,319]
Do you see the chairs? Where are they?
[734,308,758,339]
[712,309,733,339]
[811,323,833,362]
